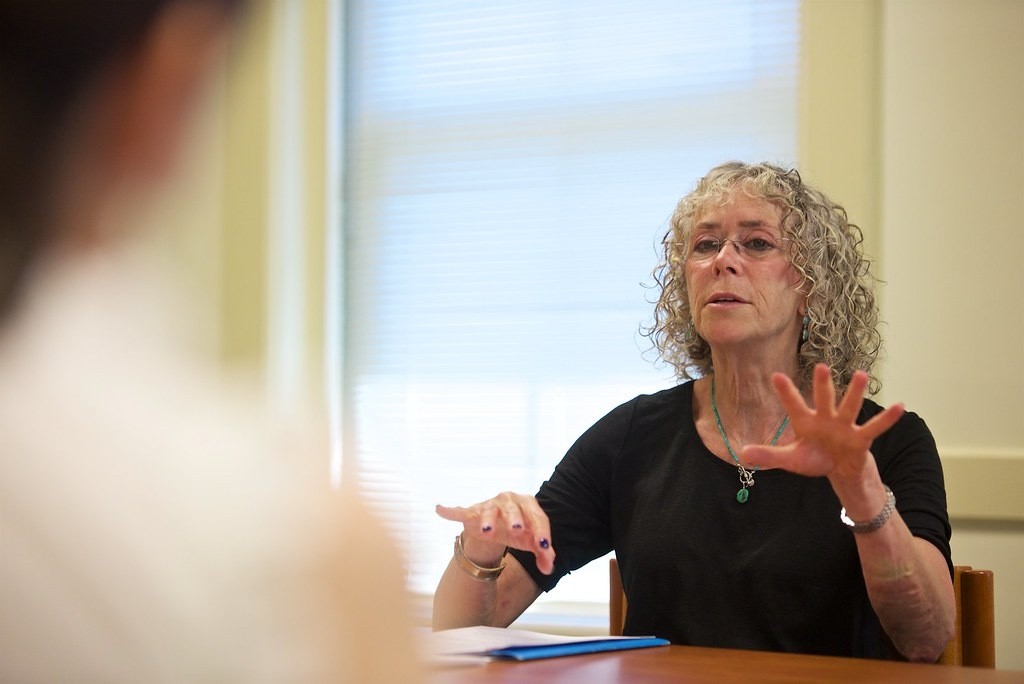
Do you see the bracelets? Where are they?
[454,532,507,580]
[840,486,896,534]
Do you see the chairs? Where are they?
[607,558,1000,672]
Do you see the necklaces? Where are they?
[710,375,806,503]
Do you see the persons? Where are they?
[431,163,955,666]
[0,0,435,684]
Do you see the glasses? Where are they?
[675,228,802,261]
